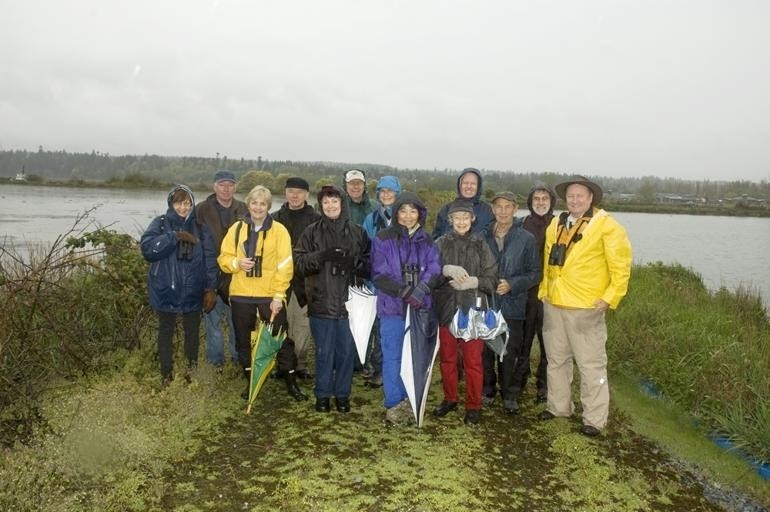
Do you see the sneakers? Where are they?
[384,406,399,426]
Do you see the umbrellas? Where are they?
[344,273,378,372]
[400,264,440,429]
[247,311,287,414]
[449,297,509,357]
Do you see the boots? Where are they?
[240,369,251,400]
[284,372,308,402]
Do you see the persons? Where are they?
[343,169,378,377]
[293,186,372,411]
[196,170,250,375]
[433,168,497,238]
[371,192,446,423]
[519,184,557,399]
[434,199,498,424]
[141,184,218,387]
[539,176,633,435]
[268,178,322,378]
[218,186,310,402]
[362,175,402,388]
[481,192,540,413]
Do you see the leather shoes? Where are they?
[364,380,384,389]
[534,409,554,421]
[465,409,481,427]
[501,399,521,415]
[316,398,330,413]
[580,424,599,436]
[335,398,351,412]
[536,390,548,403]
[432,399,458,417]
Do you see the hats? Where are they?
[447,197,474,215]
[555,174,603,206]
[344,169,365,182]
[284,176,309,191]
[491,191,517,204]
[213,169,237,184]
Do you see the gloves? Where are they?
[318,247,349,262]
[202,289,217,314]
[400,283,431,310]
[176,231,201,245]
